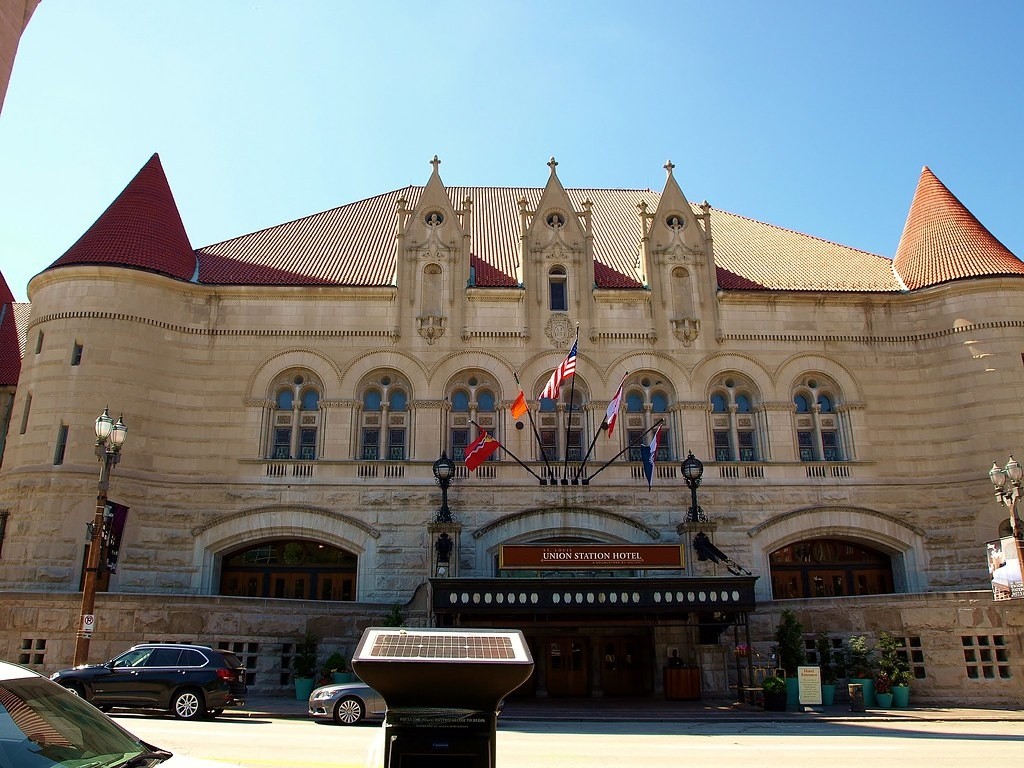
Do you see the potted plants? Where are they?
[760,676,789,711]
[876,630,915,707]
[760,608,879,707]
[293,629,319,701]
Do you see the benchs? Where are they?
[729,685,764,693]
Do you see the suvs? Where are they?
[48,644,246,720]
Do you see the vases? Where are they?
[335,672,350,684]
[877,694,893,708]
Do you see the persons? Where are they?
[668,649,683,668]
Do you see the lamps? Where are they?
[679,449,707,524]
[430,450,458,525]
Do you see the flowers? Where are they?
[874,671,893,694]
[333,666,350,674]
[317,671,332,685]
[734,642,754,659]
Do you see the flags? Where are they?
[465,428,500,471]
[537,339,576,400]
[641,425,662,492]
[606,376,627,438]
[511,390,529,420]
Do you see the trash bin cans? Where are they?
[847,683,865,712]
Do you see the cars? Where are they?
[307,683,504,726]
[0,659,242,768]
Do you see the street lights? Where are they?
[989,453,1024,586]
[74,404,126,666]
